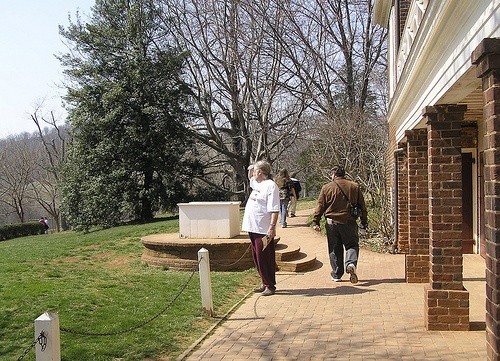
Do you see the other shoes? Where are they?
[282,225,287,227]
[262,287,276,295]
[347,264,358,284]
[331,276,341,282]
[255,284,268,292]
[289,212,295,217]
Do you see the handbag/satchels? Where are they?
[351,205,362,217]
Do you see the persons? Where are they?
[311,165,369,285]
[241,161,281,296]
[39,217,50,235]
[276,168,302,229]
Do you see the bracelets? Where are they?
[270,224,276,226]
